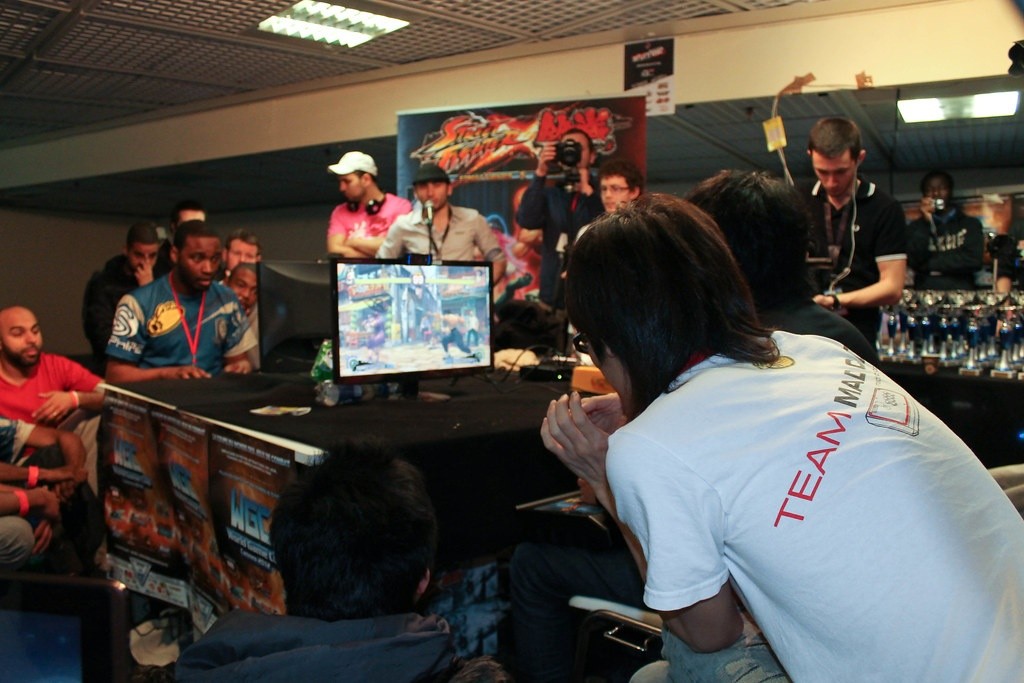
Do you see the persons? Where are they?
[540,194,1024,683]
[82,199,261,379]
[172,437,511,683]
[0,305,104,575]
[325,149,506,288]
[794,118,906,357]
[515,130,644,308]
[907,171,1024,290]
[685,168,878,365]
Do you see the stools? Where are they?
[568,596,665,683]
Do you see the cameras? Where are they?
[555,139,582,167]
[930,199,945,210]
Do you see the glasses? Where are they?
[924,185,950,196]
[597,185,629,195]
[572,332,590,354]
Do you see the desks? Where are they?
[97,368,606,683]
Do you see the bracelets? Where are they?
[826,291,838,306]
[15,491,30,517]
[26,464,40,489]
[71,391,85,412]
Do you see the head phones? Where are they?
[348,191,386,215]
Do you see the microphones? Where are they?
[424,199,433,224]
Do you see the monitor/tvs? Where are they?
[1,573,129,683]
[329,257,494,403]
[255,258,332,385]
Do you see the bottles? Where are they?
[315,379,399,407]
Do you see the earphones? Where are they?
[855,157,862,167]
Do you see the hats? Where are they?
[328,151,376,176]
[413,163,449,184]
